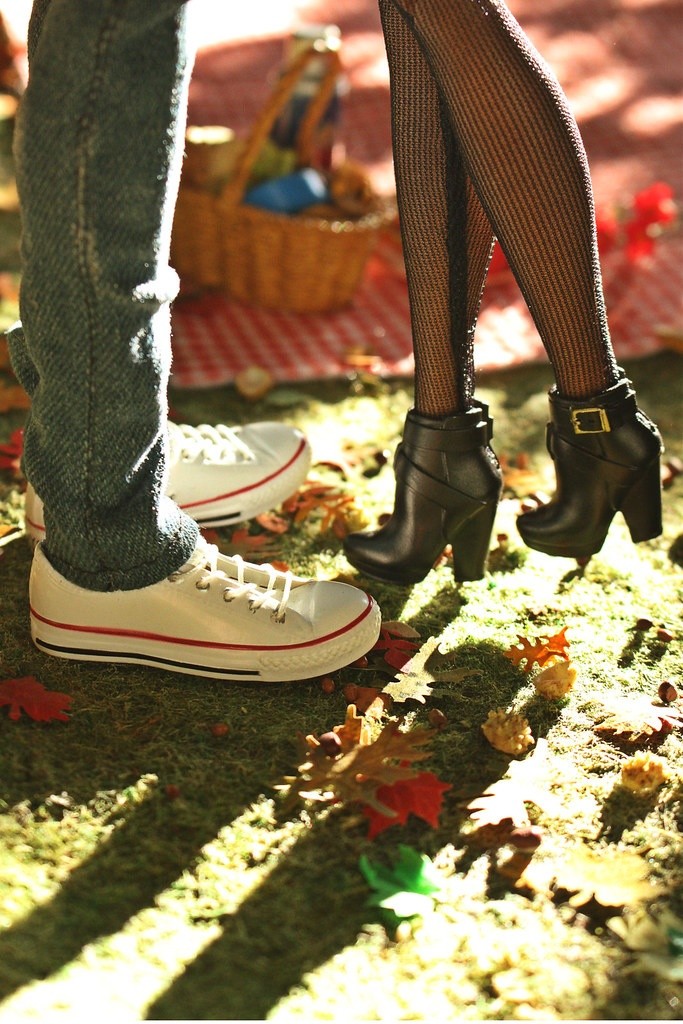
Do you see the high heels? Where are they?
[517,375,664,558]
[344,395,501,587]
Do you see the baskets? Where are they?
[172,48,393,311]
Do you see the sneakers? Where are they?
[26,534,383,682]
[16,420,312,539]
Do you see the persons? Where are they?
[10,1,384,684]
[344,0,665,586]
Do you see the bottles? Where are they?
[246,168,329,215]
[244,24,338,187]
